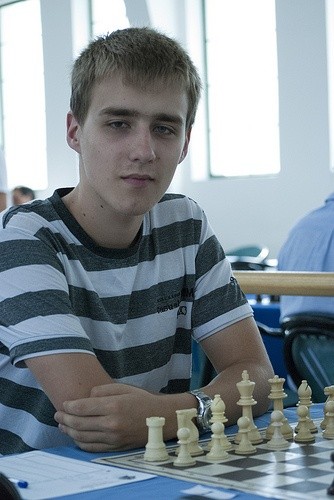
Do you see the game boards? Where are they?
[90,419,334,500]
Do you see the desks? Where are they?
[0,401,334,500]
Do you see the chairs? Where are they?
[200,312,334,403]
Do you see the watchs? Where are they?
[188,389,213,433]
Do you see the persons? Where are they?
[278,190,334,403]
[1,28,275,454]
[13,187,35,205]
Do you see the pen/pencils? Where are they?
[8,477,28,489]
